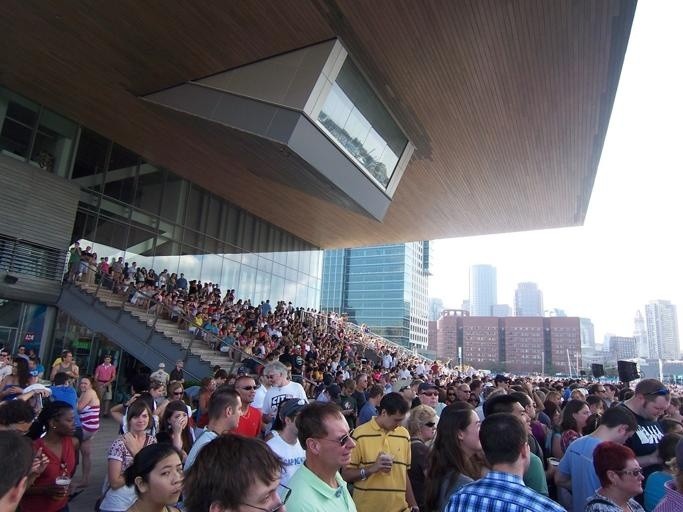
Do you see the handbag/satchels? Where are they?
[92,433,149,512]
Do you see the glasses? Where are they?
[597,390,606,393]
[423,391,439,396]
[651,388,670,396]
[364,380,367,382]
[0,354,6,357]
[448,393,456,396]
[19,348,25,352]
[614,466,642,476]
[296,397,310,406]
[243,386,258,390]
[424,422,435,428]
[173,391,182,395]
[266,374,275,379]
[464,390,472,394]
[233,483,292,512]
[312,427,355,446]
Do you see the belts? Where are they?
[97,380,109,383]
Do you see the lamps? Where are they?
[5,275,18,284]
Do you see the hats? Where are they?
[158,363,165,367]
[294,345,300,349]
